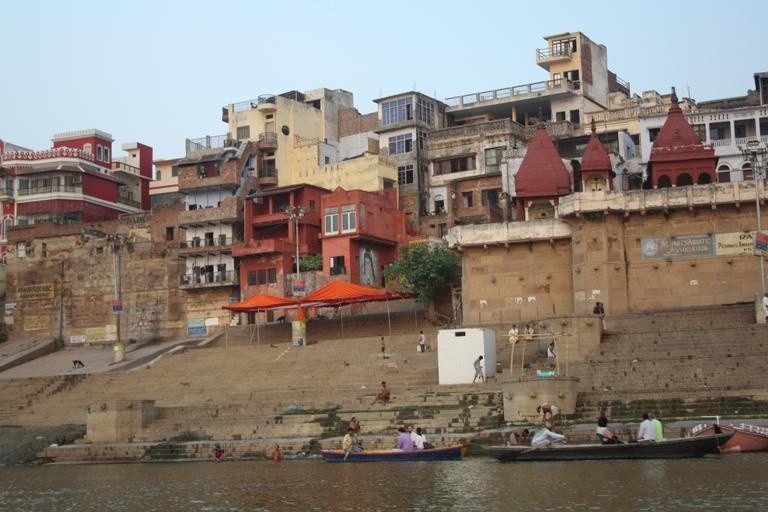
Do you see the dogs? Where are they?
[180,381,190,387]
[276,314,287,323]
[72,360,85,369]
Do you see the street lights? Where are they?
[105,232,122,343]
[285,207,305,280]
[740,140,766,298]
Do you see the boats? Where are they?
[318,443,470,462]
[480,428,737,461]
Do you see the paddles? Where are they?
[520,436,566,454]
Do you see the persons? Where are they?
[536,402,560,432]
[593,302,607,330]
[370,380,390,406]
[380,336,385,354]
[417,330,425,353]
[342,416,363,453]
[273,444,281,461]
[212,445,224,463]
[627,411,664,444]
[524,324,534,341]
[546,342,556,360]
[509,323,519,343]
[472,355,485,383]
[390,424,427,450]
[595,416,624,445]
[509,428,530,445]
